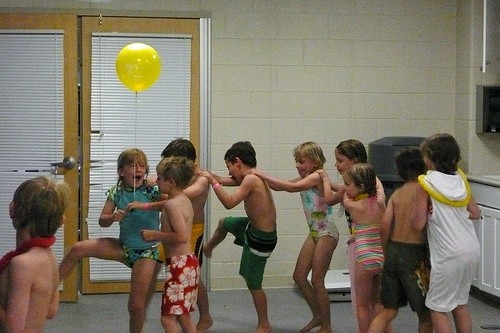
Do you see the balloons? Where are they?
[116,43,162,93]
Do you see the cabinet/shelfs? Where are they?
[469,202,500,310]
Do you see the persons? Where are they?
[126,138,210,333]
[58,147,160,333]
[198,141,278,333]
[367,149,433,333]
[246,141,339,333]
[412,132,480,333]
[317,140,385,333]
[0,177,68,333]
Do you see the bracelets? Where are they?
[144,202,149,211]
[212,183,220,190]
